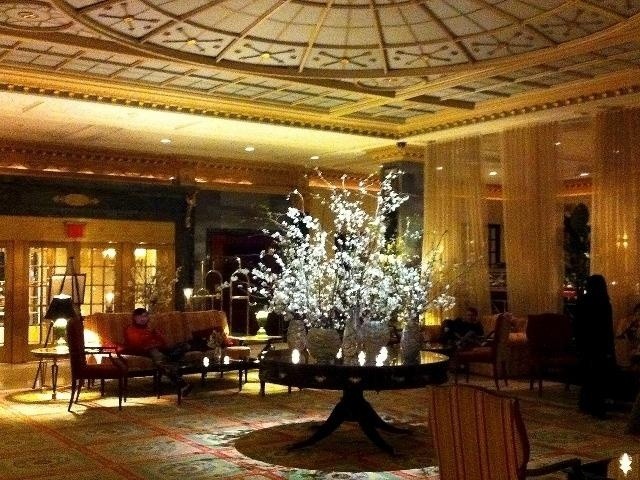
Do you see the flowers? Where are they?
[214,163,485,329]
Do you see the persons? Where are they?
[438,307,485,348]
[125,307,192,396]
[569,275,617,370]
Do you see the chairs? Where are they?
[425,385,615,479]
[58,309,261,414]
[433,309,563,391]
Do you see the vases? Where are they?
[288,319,422,364]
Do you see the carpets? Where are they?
[0,376,640,478]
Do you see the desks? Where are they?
[30,346,72,401]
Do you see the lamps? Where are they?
[44,295,76,351]
